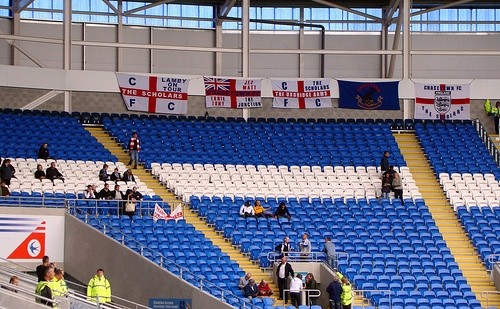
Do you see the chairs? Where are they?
[0,107,500,309]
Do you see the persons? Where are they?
[491,101,500,135]
[127,132,141,169]
[240,201,257,219]
[38,143,55,160]
[381,151,403,202]
[253,200,270,218]
[87,269,111,305]
[0,159,16,196]
[36,256,66,297]
[83,164,143,216]
[34,165,46,181]
[46,162,64,186]
[238,233,352,309]
[35,267,56,307]
[1,276,19,293]
[275,202,291,218]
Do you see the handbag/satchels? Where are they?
[125,194,135,212]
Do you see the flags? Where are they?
[204,76,264,108]
[115,73,191,114]
[338,80,400,110]
[414,82,471,120]
[270,79,332,108]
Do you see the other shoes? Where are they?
[285,301,288,304]
[134,165,137,169]
[277,297,282,300]
[128,164,131,166]
